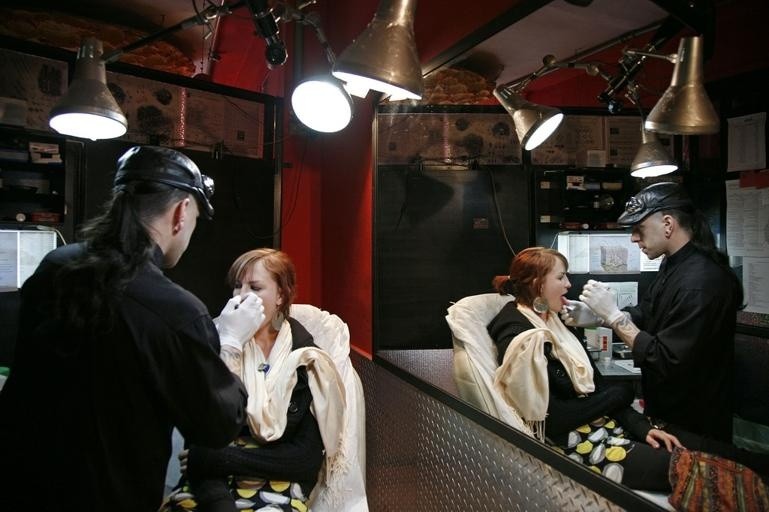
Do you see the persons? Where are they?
[486,246,768,492]
[0,145,266,512]
[158,247,326,512]
[617,182,743,443]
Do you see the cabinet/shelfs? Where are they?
[537,167,633,230]
[0,126,77,230]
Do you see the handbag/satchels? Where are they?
[668,446,768,512]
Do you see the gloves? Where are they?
[579,279,623,324]
[560,299,606,328]
[216,293,265,350]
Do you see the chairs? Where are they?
[289,303,367,512]
[446,293,676,511]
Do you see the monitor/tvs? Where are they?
[557,230,666,277]
[0,223,60,299]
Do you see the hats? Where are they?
[115,146,215,223]
[616,182,689,225]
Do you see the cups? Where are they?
[598,328,612,360]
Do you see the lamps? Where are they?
[331,0,422,105]
[493,36,721,178]
[290,20,355,137]
[50,32,130,138]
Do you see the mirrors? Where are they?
[371,0,769,512]
